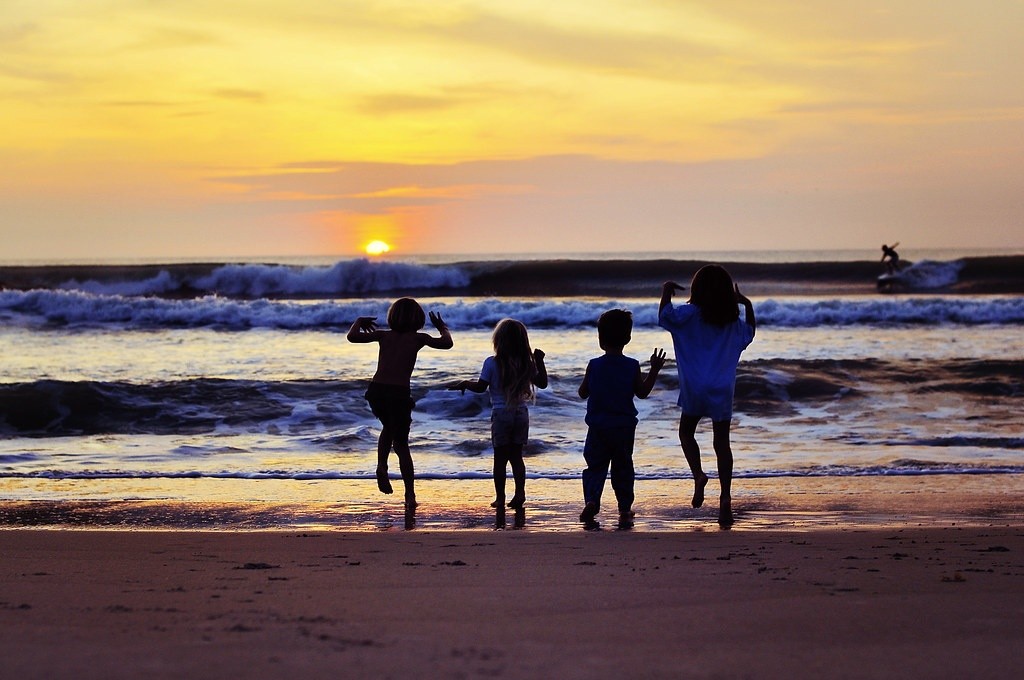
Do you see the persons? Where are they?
[346,297,454,509]
[659,265,756,521]
[880,243,902,275]
[579,309,667,519]
[448,318,548,506]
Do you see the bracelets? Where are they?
[440,326,449,335]
